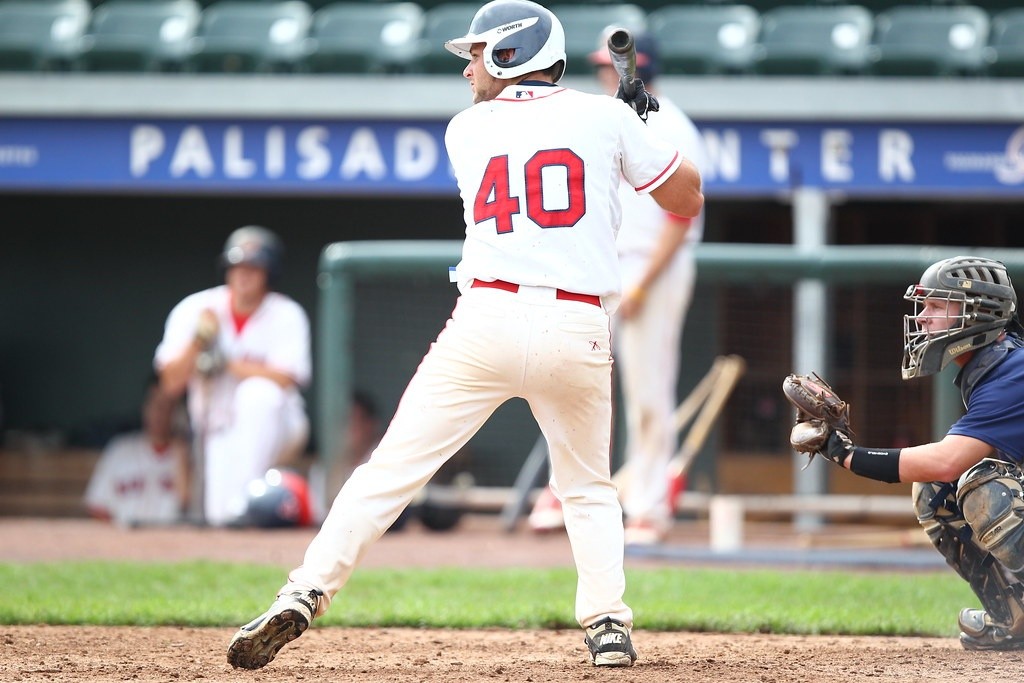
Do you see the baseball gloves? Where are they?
[782,370,849,455]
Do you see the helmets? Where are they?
[900,256,1016,380]
[588,24,656,77]
[219,225,280,277]
[443,0,567,84]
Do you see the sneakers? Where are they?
[228,587,323,669]
[961,627,1024,651]
[584,616,636,667]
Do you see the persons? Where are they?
[83,225,472,532]
[783,255,1024,649]
[227,0,703,669]
[529,24,702,547]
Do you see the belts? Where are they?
[470,278,602,307]
